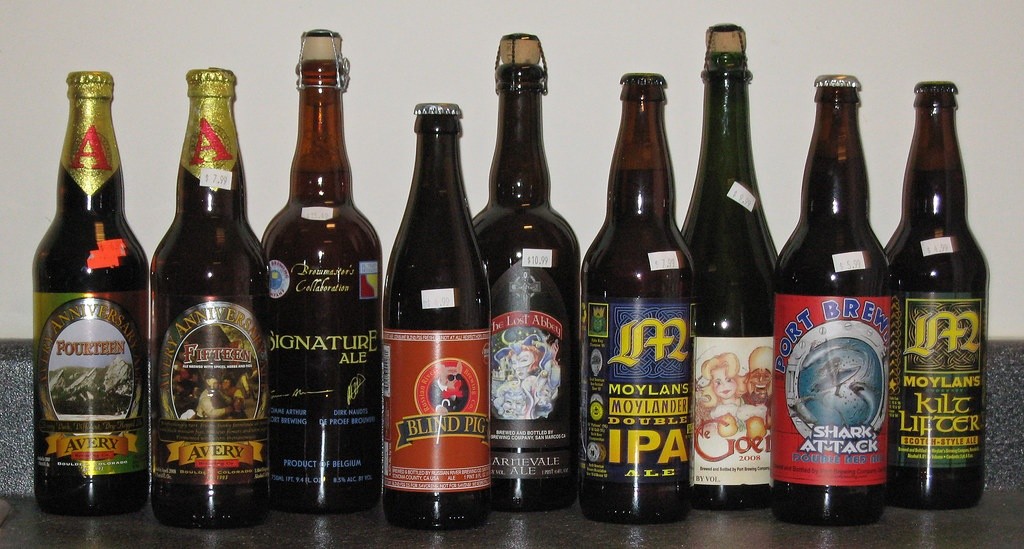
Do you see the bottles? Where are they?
[579,73,696,525]
[882,81,991,509]
[150,68,270,532]
[769,75,891,525]
[260,27,382,514]
[471,34,581,513]
[678,24,779,510]
[381,104,492,529]
[31,71,149,517]
